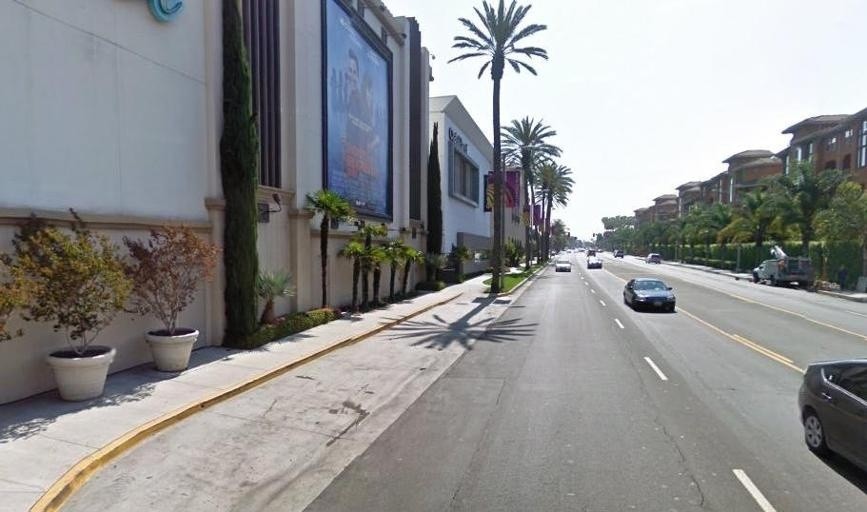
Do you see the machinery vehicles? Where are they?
[752,244,814,288]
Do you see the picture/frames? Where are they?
[321,0,394,223]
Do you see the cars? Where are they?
[586,248,603,269]
[798,358,867,473]
[556,257,571,272]
[623,277,676,312]
[613,249,661,264]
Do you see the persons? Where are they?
[838,264,848,290]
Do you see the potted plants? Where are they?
[1,207,132,402]
[115,219,223,372]
